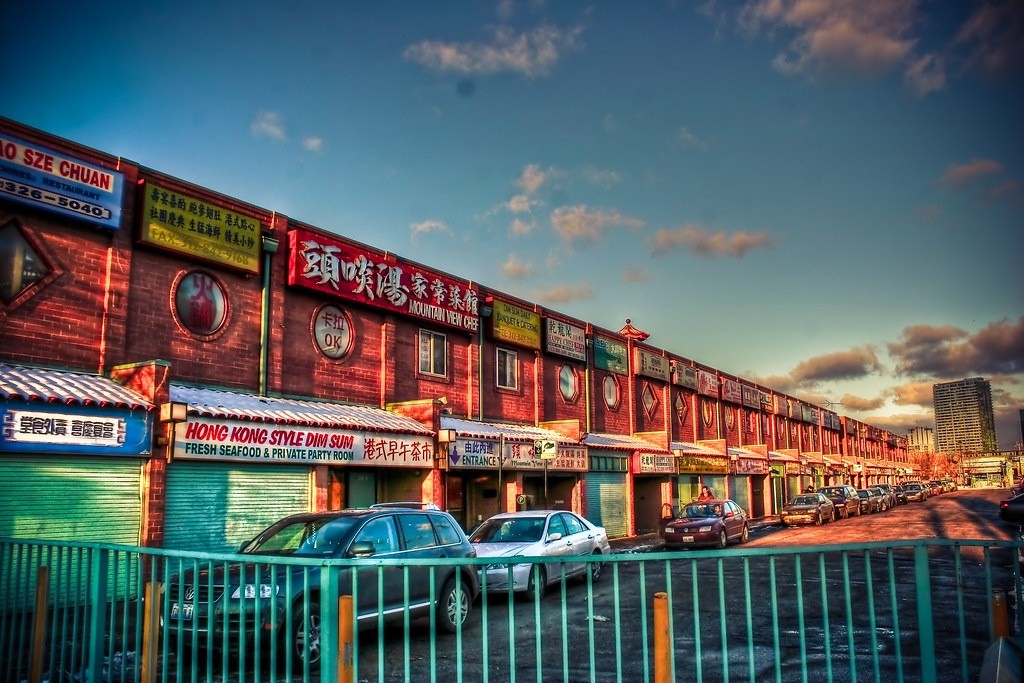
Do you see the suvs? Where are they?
[155,500,480,670]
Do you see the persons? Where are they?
[698,486,714,500]
[805,485,814,493]
[714,504,721,516]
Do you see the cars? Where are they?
[868,487,891,512]
[890,485,908,506]
[999,493,1024,529]
[856,489,880,515]
[658,497,751,549]
[924,477,958,497]
[780,492,835,526]
[467,510,612,600]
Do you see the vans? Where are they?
[815,484,862,519]
[869,483,898,508]
[903,483,930,502]
